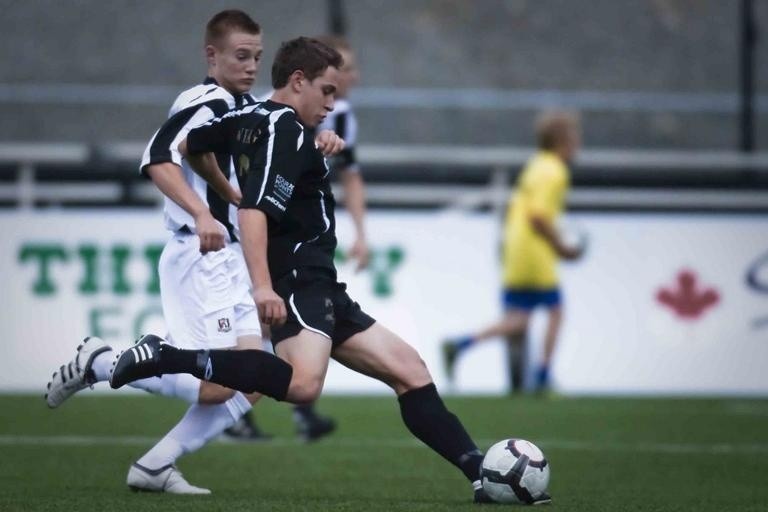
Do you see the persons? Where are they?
[42,9,350,502]
[111,38,549,506]
[221,38,368,440]
[441,103,587,396]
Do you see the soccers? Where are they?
[479,438,550,504]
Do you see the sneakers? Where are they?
[109,334,172,389]
[471,479,551,505]
[45,338,112,409]
[292,414,334,441]
[127,461,211,496]
[222,417,267,438]
